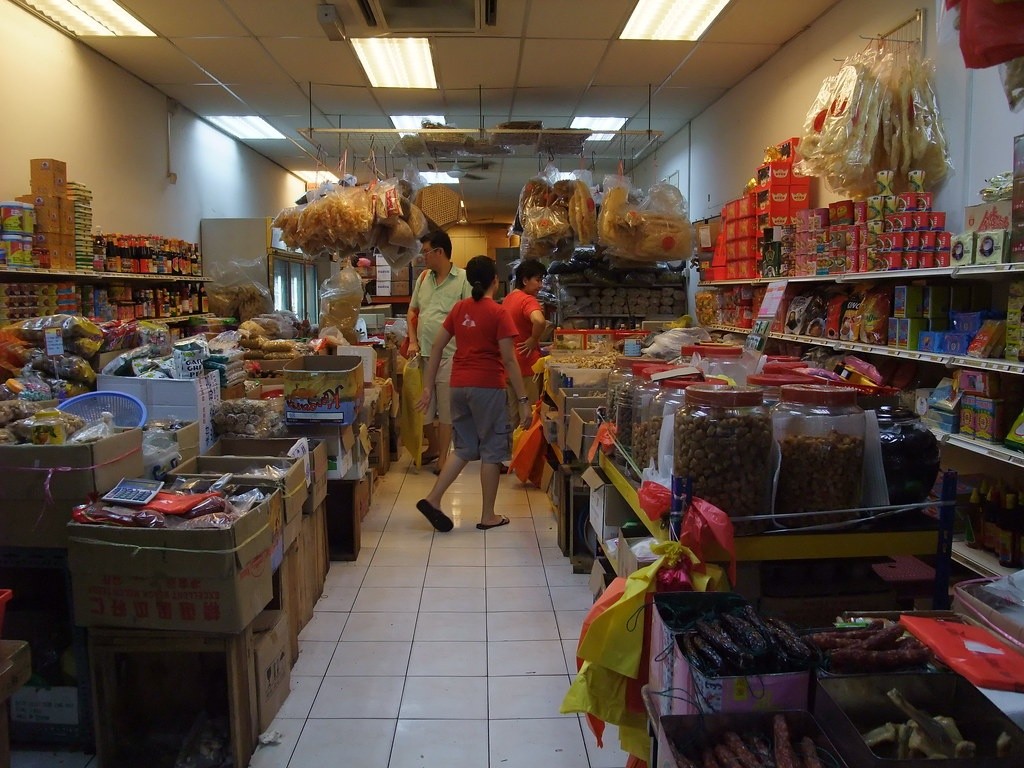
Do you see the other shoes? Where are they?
[499,462,513,474]
[414,449,440,465]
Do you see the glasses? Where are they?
[422,248,438,257]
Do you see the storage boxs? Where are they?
[375,255,428,297]
[0,305,394,733]
[540,328,1024,651]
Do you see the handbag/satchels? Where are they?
[398,356,424,470]
[560,553,730,768]
[507,400,543,483]
[399,269,429,360]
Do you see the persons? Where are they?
[407,230,472,477]
[500,259,549,473]
[416,255,533,532]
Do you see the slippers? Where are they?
[416,498,454,532]
[476,514,510,530]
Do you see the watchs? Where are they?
[518,396,528,404]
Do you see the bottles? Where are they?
[91,226,211,320]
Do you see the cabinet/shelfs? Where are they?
[556,281,686,328]
[697,260,1024,581]
[0,266,216,326]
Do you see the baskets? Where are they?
[55,391,148,429]
[416,184,462,232]
